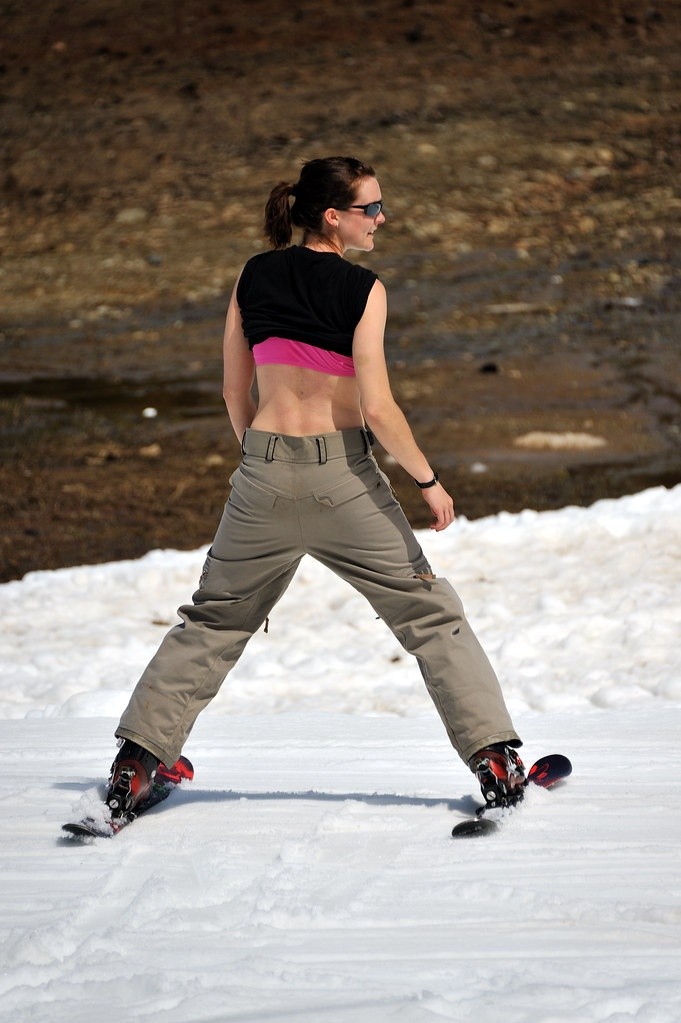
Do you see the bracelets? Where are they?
[414,470,439,489]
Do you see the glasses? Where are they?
[350,200,385,217]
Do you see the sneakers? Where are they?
[105,741,158,809]
[470,741,525,802]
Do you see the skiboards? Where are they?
[55,733,573,841]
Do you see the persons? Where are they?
[58,152,575,840]
